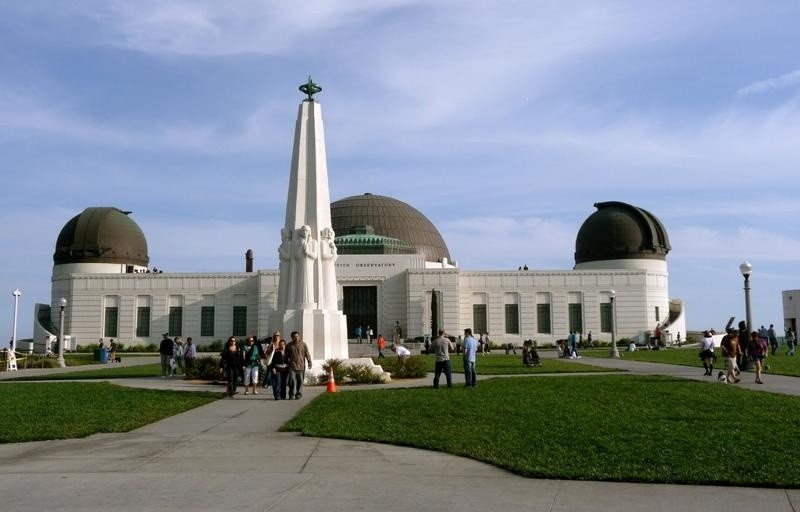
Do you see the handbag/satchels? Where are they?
[170,359,177,370]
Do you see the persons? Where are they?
[460,329,478,388]
[98,338,104,348]
[429,328,456,389]
[355,320,594,364]
[320,228,338,309]
[135,266,164,274]
[294,225,317,303]
[699,316,795,384]
[218,331,312,400]
[627,339,668,352]
[44,347,52,359]
[109,338,116,363]
[158,333,196,380]
[524,265,528,271]
[9,336,13,350]
[276,228,292,303]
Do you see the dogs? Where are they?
[718,371,728,384]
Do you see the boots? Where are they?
[703,363,713,376]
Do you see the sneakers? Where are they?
[728,379,740,383]
[275,395,300,400]
[756,380,763,384]
[162,373,186,379]
[245,392,259,395]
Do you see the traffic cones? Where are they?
[326,366,336,393]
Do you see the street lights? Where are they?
[739,260,755,370]
[607,290,620,359]
[55,297,67,368]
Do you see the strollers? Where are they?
[523,340,542,366]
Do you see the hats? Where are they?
[162,333,169,336]
[750,331,758,336]
